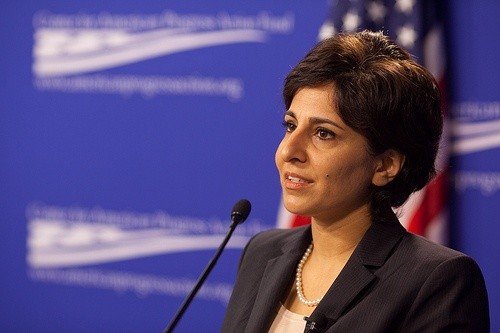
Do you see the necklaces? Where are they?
[296,244,321,306]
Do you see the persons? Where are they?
[220,30,490,333]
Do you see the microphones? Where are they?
[164,199,252,333]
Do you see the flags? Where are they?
[276,0,447,244]
